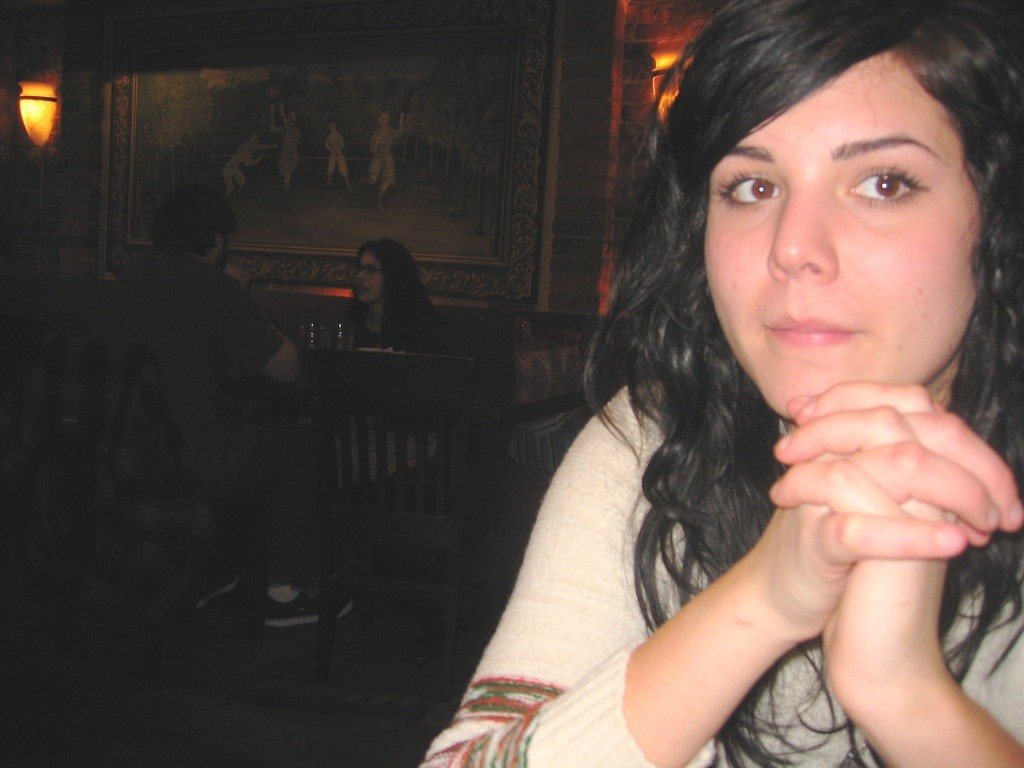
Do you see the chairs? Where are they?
[315,346,478,663]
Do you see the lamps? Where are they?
[650,51,685,119]
[18,79,57,147]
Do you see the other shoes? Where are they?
[261,585,323,628]
[193,563,237,607]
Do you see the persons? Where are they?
[98,184,456,627]
[418,0,1024,768]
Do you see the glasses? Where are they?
[355,264,384,274]
